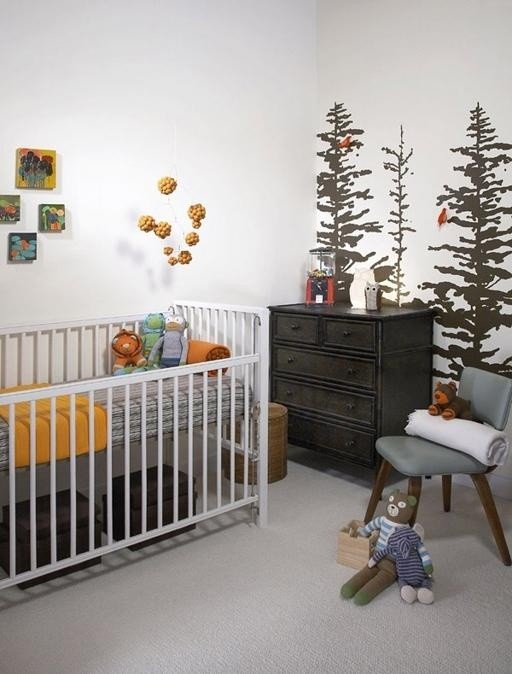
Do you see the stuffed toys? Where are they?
[365,282,382,311]
[148,313,189,368]
[368,527,434,603]
[111,329,147,373]
[341,489,432,607]
[428,381,475,419]
[113,312,165,376]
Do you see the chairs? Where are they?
[364,367,512,565]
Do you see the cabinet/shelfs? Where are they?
[270,300,434,498]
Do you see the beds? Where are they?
[0,303,270,589]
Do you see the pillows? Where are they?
[188,339,230,375]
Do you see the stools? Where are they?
[224,402,288,485]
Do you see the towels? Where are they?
[402,408,507,469]
